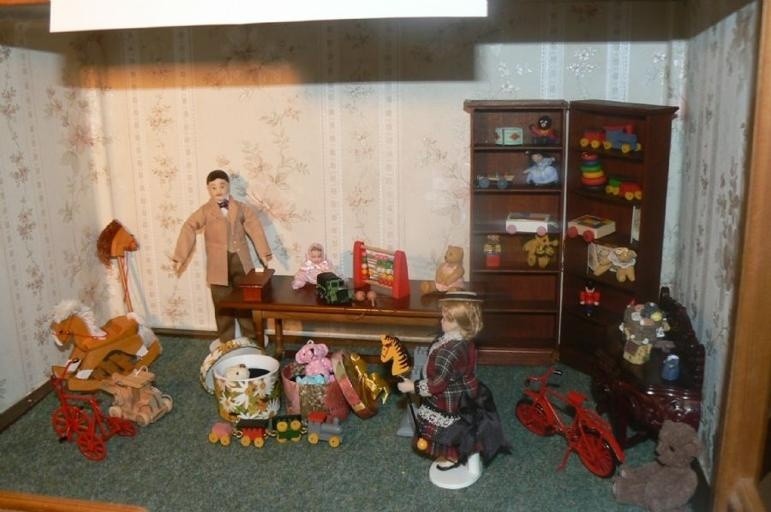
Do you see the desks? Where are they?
[597,328,705,451]
[217,272,473,356]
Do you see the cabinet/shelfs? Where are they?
[561,99,681,376]
[463,97,569,366]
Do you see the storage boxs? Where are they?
[282,356,352,421]
[211,353,282,421]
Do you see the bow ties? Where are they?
[215,199,229,208]
